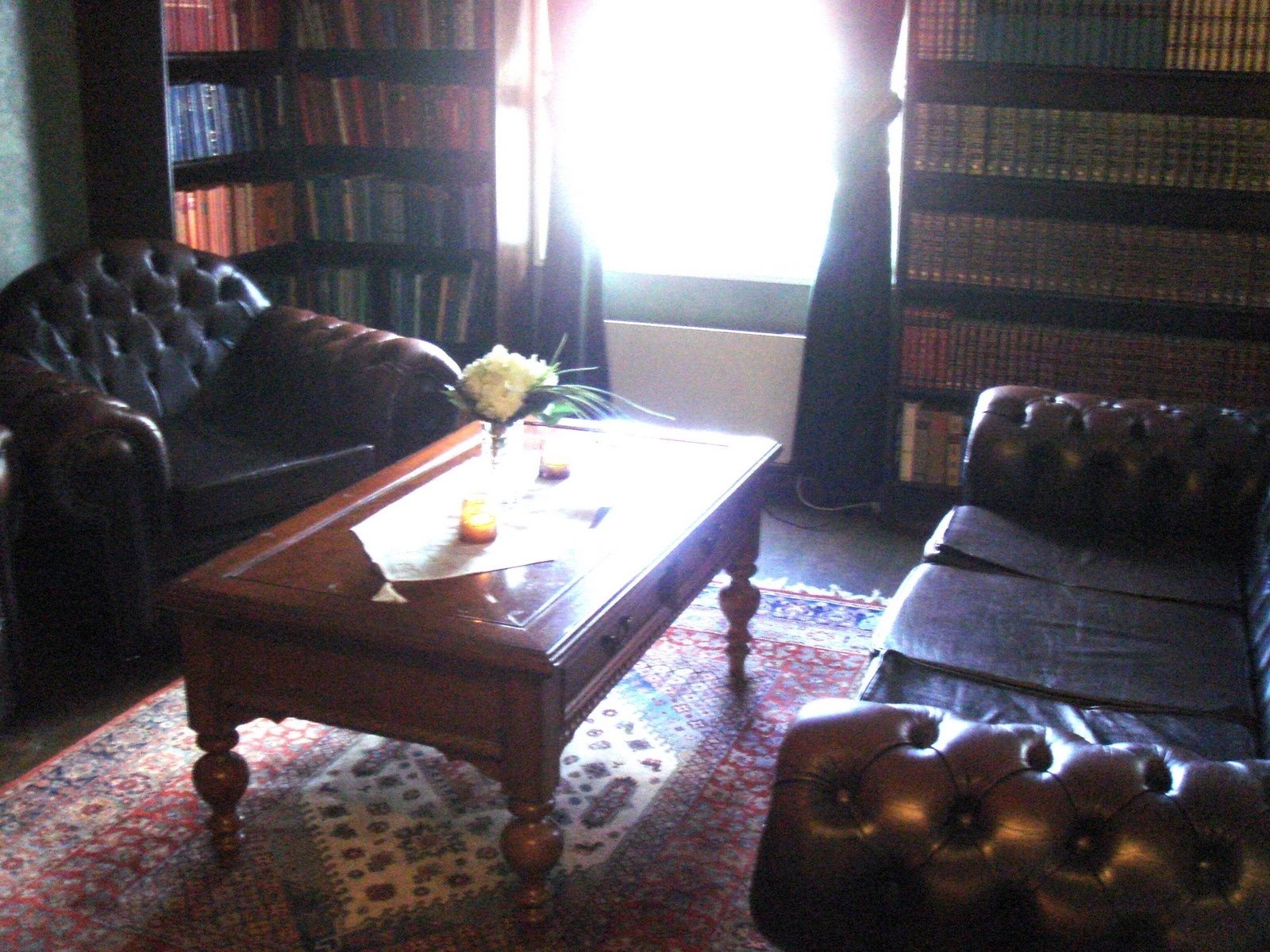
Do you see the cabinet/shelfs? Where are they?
[74,0,484,348]
[882,0,1270,523]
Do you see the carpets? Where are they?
[1,575,890,951]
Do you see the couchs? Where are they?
[749,384,1270,952]
[0,239,460,685]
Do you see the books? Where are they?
[896,400,963,487]
[909,101,1270,189]
[169,75,491,164]
[906,211,1270,308]
[253,259,480,346]
[162,1,496,52]
[898,310,1270,412]
[917,0,1270,71]
[173,172,494,258]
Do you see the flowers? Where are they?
[441,330,676,425]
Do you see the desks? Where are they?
[135,413,782,938]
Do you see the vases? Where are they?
[459,422,507,544]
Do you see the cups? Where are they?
[458,491,497,545]
[538,440,571,480]
